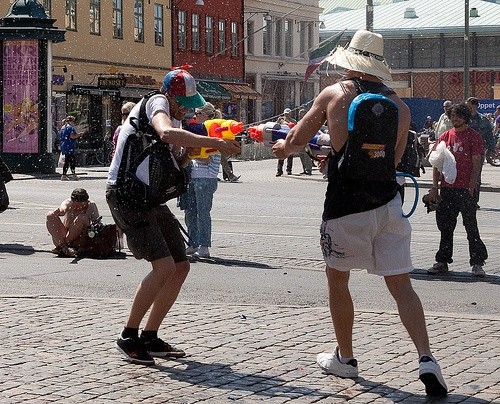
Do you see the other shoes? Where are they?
[72,174,79,181]
[276,171,283,177]
[185,245,199,255]
[198,245,211,258]
[52,242,76,257]
[60,175,73,184]
[227,171,241,183]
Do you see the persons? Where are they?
[106,70,243,366]
[112,101,137,249]
[55,115,84,181]
[186,102,222,257]
[427,101,488,276]
[272,29,448,397]
[235,120,256,161]
[484,105,500,159]
[0,155,13,213]
[298,109,314,176]
[435,100,454,142]
[275,108,296,177]
[212,109,241,183]
[464,97,493,210]
[46,188,99,258]
[396,116,437,177]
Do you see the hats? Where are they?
[71,188,89,202]
[121,102,135,114]
[163,69,207,108]
[324,29,395,83]
[283,108,291,114]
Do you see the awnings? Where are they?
[252,121,265,161]
[69,84,271,100]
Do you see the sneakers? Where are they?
[115,326,155,365]
[419,354,448,397]
[426,262,448,276]
[472,264,486,277]
[139,330,185,357]
[316,345,358,377]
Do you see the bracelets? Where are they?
[432,186,438,188]
[470,180,479,185]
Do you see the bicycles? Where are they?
[486,144,500,167]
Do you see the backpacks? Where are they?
[114,89,188,210]
[336,77,399,204]
[83,221,121,259]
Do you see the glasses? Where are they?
[193,112,202,116]
